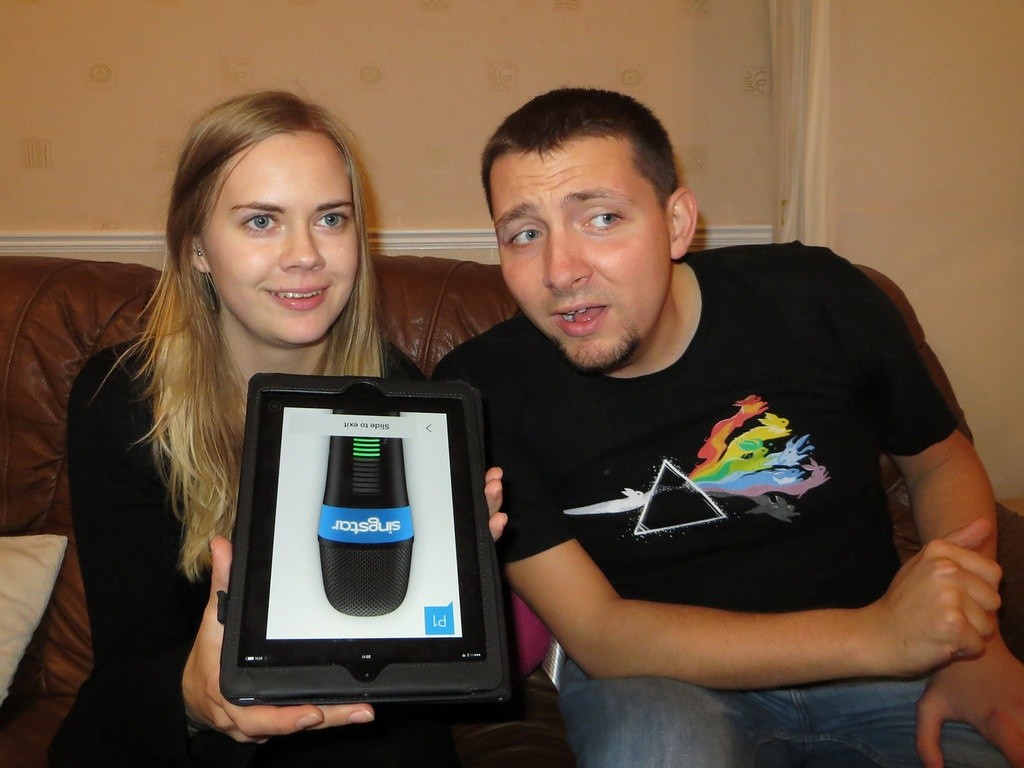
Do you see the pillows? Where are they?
[0,532,68,706]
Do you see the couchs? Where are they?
[0,254,920,768]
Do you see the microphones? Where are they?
[314,434,413,616]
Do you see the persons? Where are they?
[427,87,1024,768]
[49,93,575,768]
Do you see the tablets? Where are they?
[214,368,511,708]
